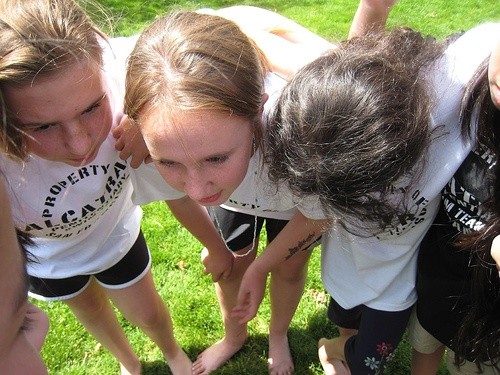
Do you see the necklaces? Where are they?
[212,143,258,257]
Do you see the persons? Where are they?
[0,0,500,375]
